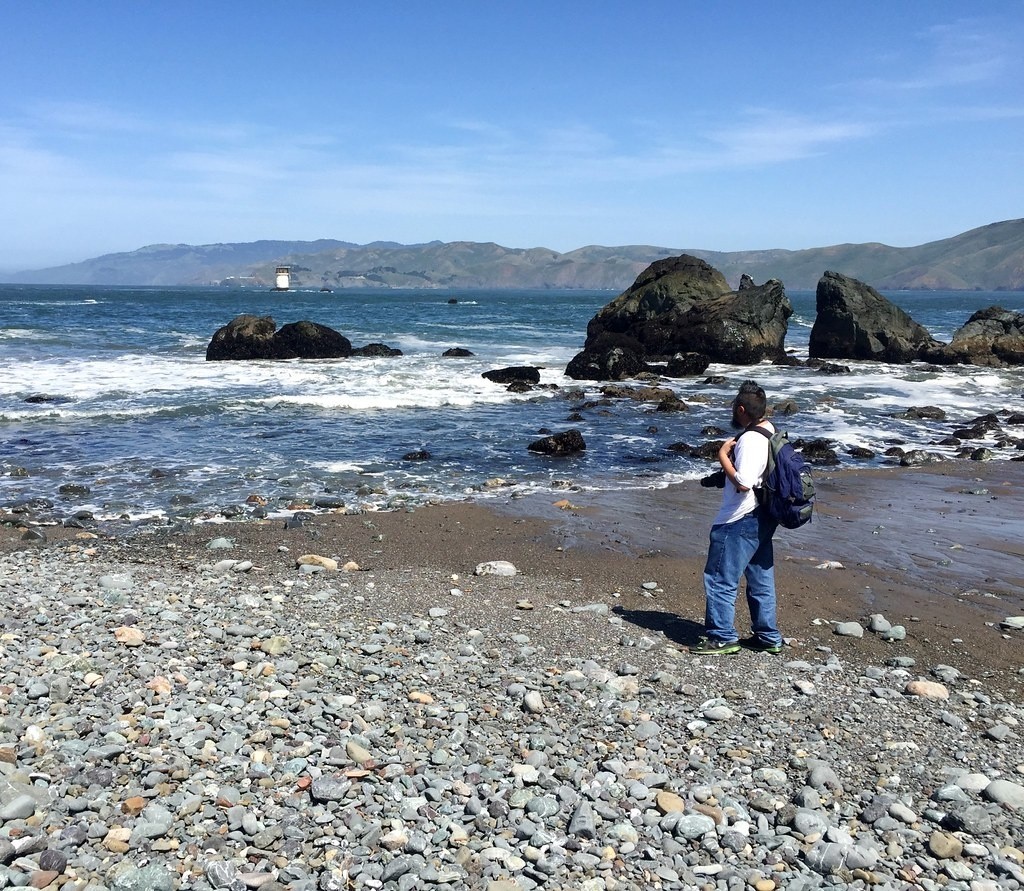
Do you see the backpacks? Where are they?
[732,426,815,529]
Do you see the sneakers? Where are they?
[738,634,782,652]
[689,638,741,655]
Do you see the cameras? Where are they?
[700,470,726,488]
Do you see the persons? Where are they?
[690,379,784,654]
